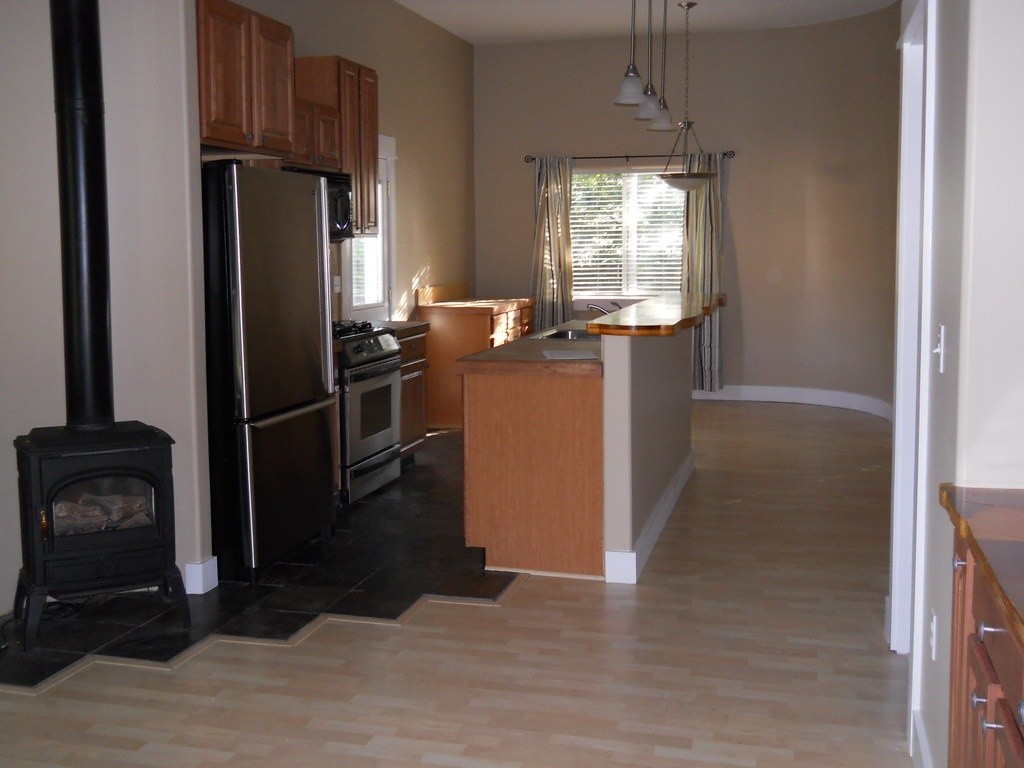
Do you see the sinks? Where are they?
[529,326,601,341]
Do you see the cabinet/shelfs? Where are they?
[196,0,294,158]
[937,480,1024,768]
[417,296,536,430]
[296,54,379,240]
[368,320,431,462]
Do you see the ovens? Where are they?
[339,354,402,504]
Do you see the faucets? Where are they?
[587,304,611,315]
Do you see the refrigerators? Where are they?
[199,162,342,569]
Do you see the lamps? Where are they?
[655,2,717,191]
[647,0,679,131]
[614,0,649,107]
[633,0,659,120]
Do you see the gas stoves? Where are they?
[332,318,400,365]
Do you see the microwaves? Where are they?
[282,166,355,243]
